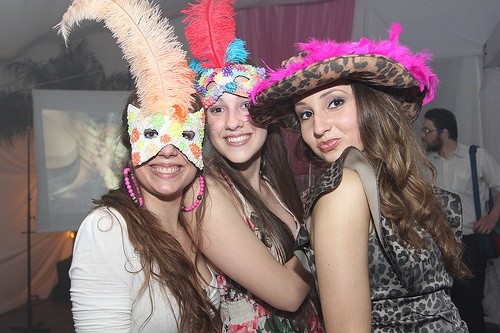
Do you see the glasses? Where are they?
[423,127,439,133]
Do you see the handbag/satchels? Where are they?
[476,230,500,259]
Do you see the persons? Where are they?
[179,56,325,333]
[421,108,500,333]
[69,88,222,333]
[292,37,473,333]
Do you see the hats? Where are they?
[248,49,427,131]
